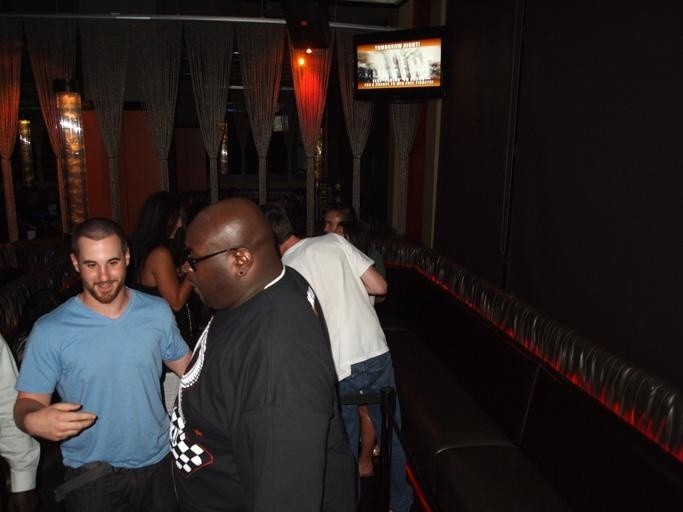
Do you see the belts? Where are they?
[54,462,129,503]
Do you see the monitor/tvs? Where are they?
[353,26,445,105]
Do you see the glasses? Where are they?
[187,249,237,272]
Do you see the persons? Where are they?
[168,197,361,512]
[132,190,194,345]
[323,204,388,304]
[335,220,370,256]
[259,202,415,512]
[13,214,194,512]
[0,333,42,512]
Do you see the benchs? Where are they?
[378,244,682,512]
